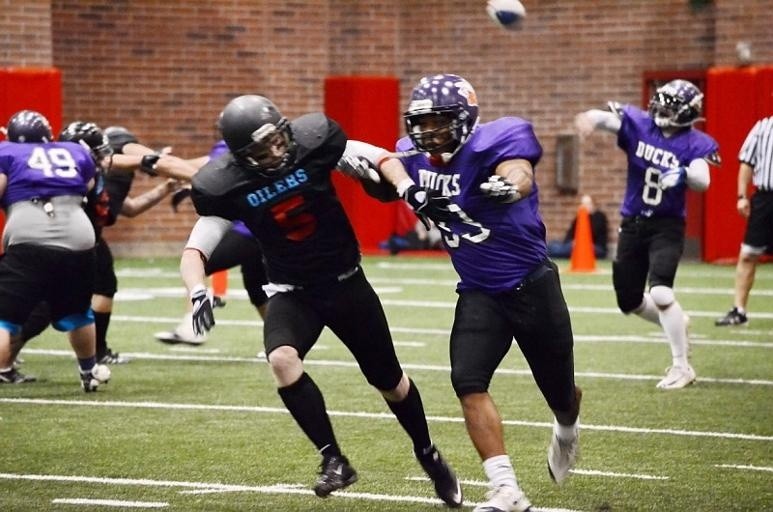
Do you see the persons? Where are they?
[313,66,586,512]
[712,106,772,330]
[176,91,469,511]
[0,111,274,394]
[568,71,704,394]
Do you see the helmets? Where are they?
[649,79,706,128]
[404,74,480,153]
[9,110,53,143]
[58,122,113,161]
[220,94,298,182]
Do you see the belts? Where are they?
[515,266,549,292]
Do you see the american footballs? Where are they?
[487,0,526,31]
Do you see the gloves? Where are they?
[658,168,686,192]
[401,185,460,231]
[191,290,225,336]
[470,175,521,206]
[102,126,137,155]
[334,155,380,184]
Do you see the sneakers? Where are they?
[715,307,748,326]
[0,362,36,383]
[414,439,463,506]
[655,366,696,389]
[79,364,111,392]
[547,419,577,484]
[315,455,358,496]
[472,485,532,512]
[155,316,207,343]
[96,348,131,364]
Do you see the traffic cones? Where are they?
[569,205,597,274]
